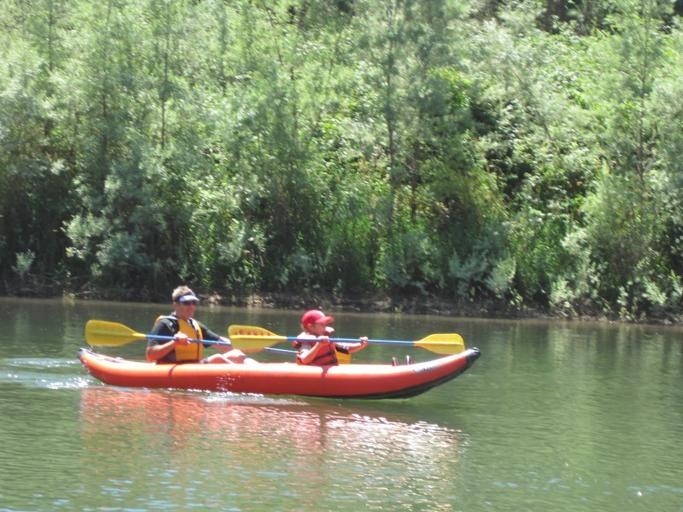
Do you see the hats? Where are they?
[172,291,200,303]
[301,309,334,328]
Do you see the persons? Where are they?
[146,284,262,365]
[291,308,368,364]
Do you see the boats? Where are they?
[75,346,482,400]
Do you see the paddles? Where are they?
[84,320,351,364]
[229,324,465,355]
